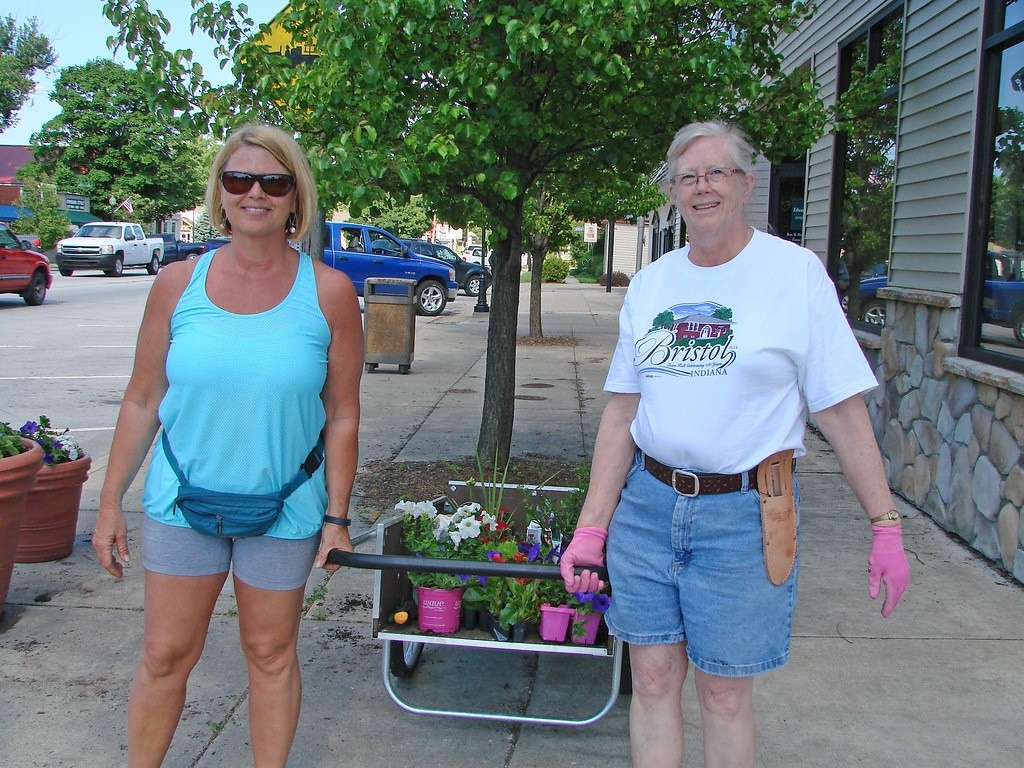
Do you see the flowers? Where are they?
[20,414,83,465]
[394,427,611,641]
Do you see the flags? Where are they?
[123,196,133,212]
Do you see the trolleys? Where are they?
[323,480,632,726]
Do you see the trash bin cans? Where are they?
[363,276,418,374]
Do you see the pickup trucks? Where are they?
[56,221,164,277]
[144,233,207,266]
[203,220,459,316]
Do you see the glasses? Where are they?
[671,167,745,186]
[219,170,298,197]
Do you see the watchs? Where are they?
[871,510,899,523]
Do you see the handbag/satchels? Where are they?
[177,485,284,537]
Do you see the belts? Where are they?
[642,453,795,498]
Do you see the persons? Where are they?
[93,125,365,768]
[561,122,910,768]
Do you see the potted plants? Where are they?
[0,421,45,614]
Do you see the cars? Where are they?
[461,244,493,266]
[0,222,53,305]
[373,237,493,297]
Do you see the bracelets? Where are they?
[324,515,352,525]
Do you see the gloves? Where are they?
[561,527,609,593]
[867,523,911,618]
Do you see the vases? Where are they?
[535,603,576,643]
[417,587,462,633]
[570,612,602,645]
[489,615,510,642]
[479,611,489,631]
[15,454,92,563]
[464,609,476,630]
[510,623,527,642]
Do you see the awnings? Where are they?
[0,205,104,225]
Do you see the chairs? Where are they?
[346,236,363,253]
